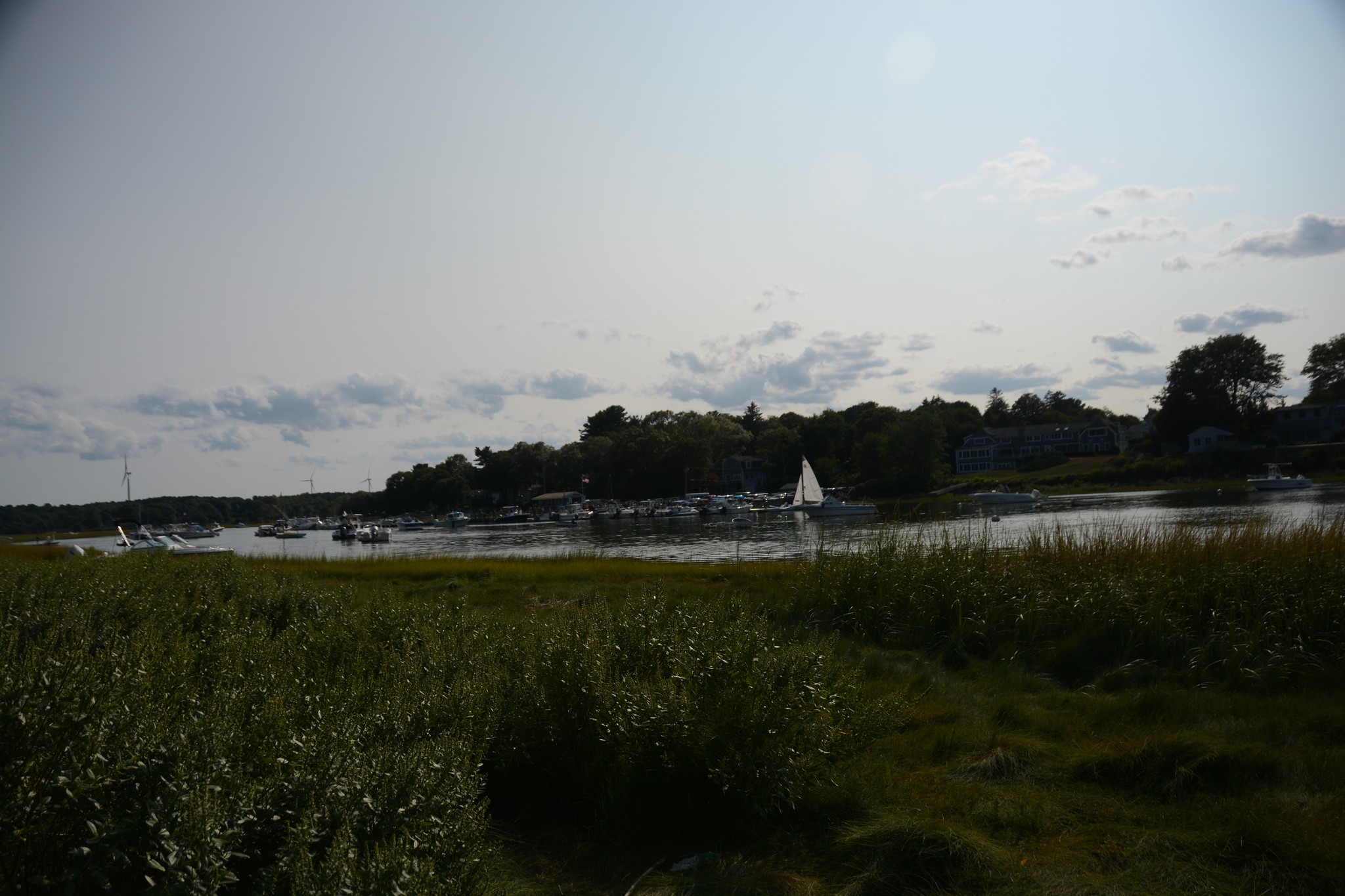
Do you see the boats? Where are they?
[968,493,1039,504]
[253,516,424,542]
[1246,479,1312,489]
[557,502,801,521]
[43,540,61,545]
[431,511,470,528]
[116,522,235,555]
[731,517,753,528]
[493,505,531,523]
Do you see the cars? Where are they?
[656,491,797,506]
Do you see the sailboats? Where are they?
[793,454,880,517]
